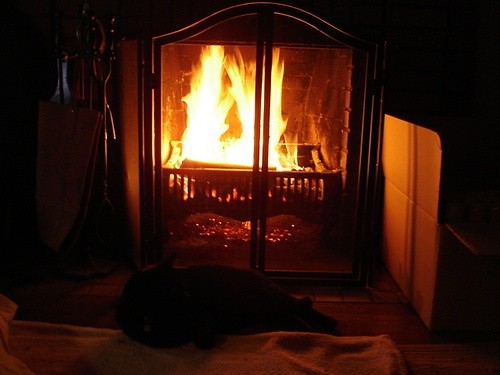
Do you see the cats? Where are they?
[114,250,339,351]
